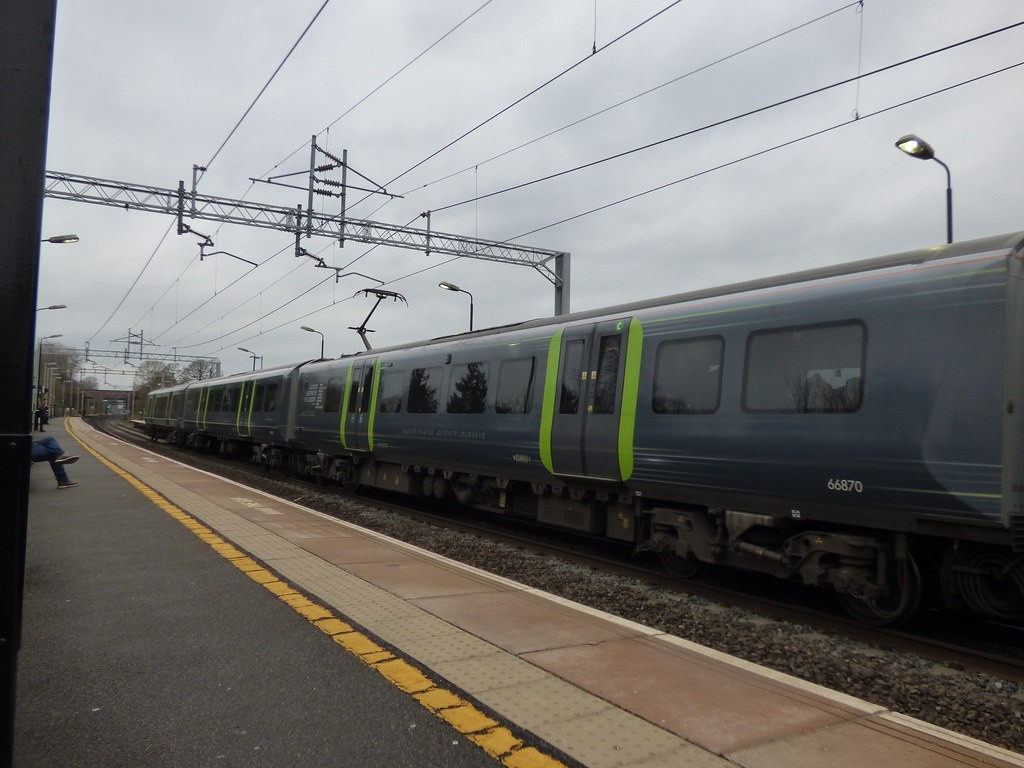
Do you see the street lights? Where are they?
[237,347,255,372]
[62,381,85,417]
[438,281,474,332]
[299,326,324,359]
[249,356,263,370]
[33,334,63,432]
[895,135,953,245]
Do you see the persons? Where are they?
[38,399,48,431]
[30,437,79,488]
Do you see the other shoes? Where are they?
[39,430,46,432]
[43,422,48,424]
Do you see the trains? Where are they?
[144,233,1024,629]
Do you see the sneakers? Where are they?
[54,454,79,464]
[57,479,78,487]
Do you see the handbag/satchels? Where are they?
[36,407,41,417]
